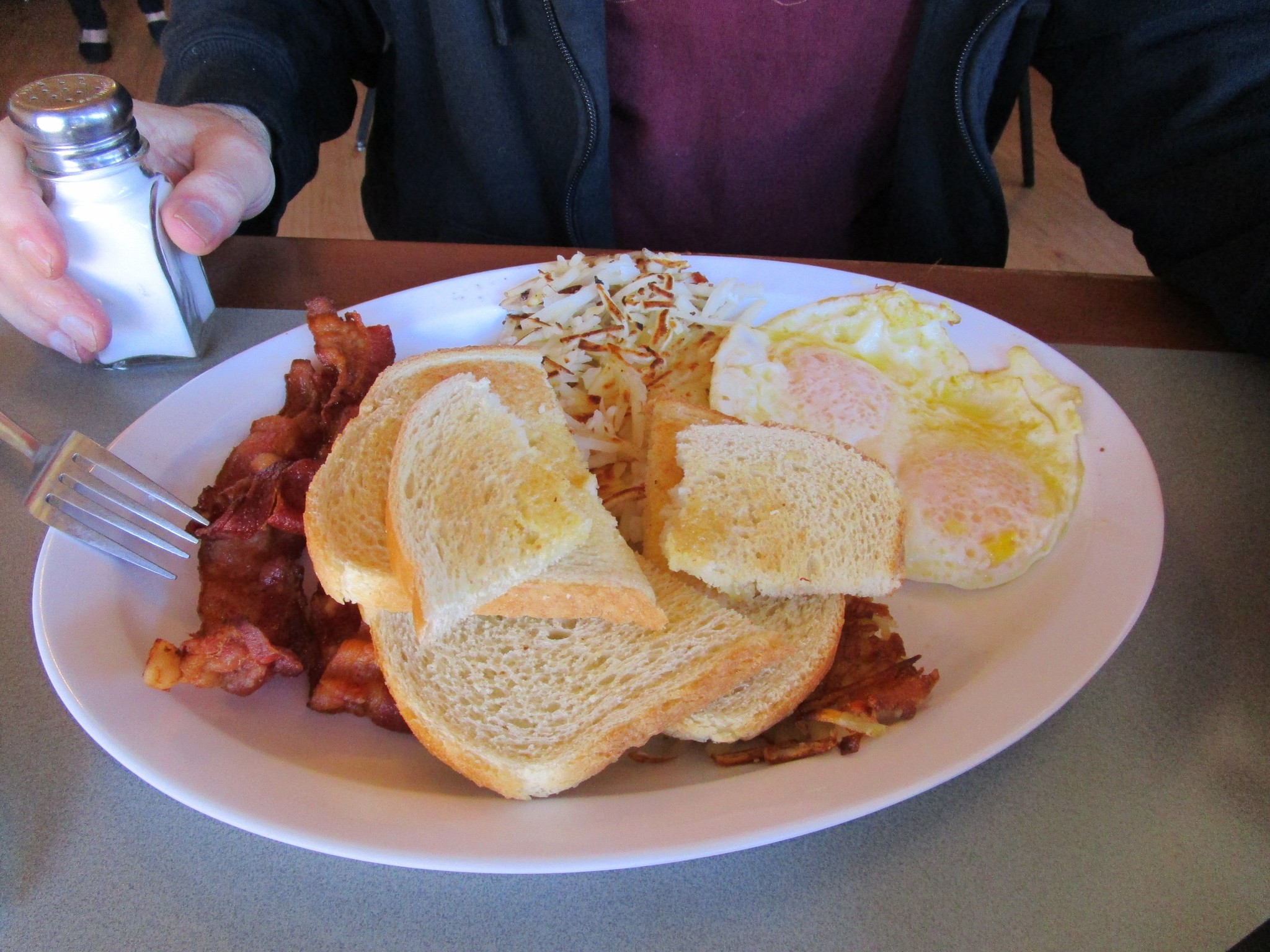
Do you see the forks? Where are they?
[0,411,211,581]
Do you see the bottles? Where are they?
[7,71,220,372]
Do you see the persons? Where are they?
[0,0,1270,367]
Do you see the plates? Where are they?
[30,252,1165,876]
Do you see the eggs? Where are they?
[709,284,1085,589]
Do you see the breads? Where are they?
[303,345,781,800]
[643,397,905,744]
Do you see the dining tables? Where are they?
[0,236,1270,952]
